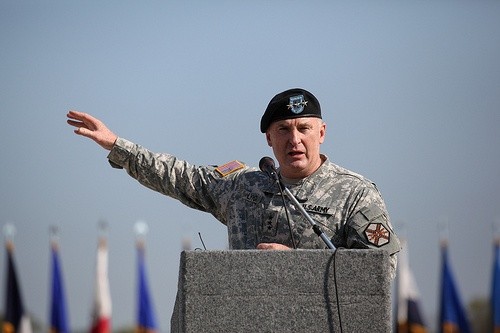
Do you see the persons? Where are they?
[66,88,401,279]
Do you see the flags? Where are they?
[46,242,70,333]
[135,239,158,333]
[437,249,473,333]
[88,235,112,333]
[0,239,25,333]
[397,236,428,333]
[488,244,500,333]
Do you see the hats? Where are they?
[260,88,322,133]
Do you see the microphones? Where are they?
[259,157,280,184]
